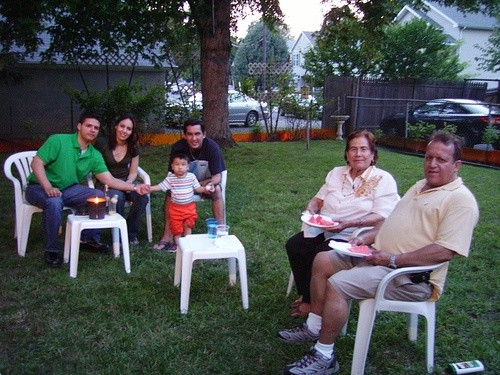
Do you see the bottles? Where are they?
[109,195,118,214]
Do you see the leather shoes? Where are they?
[80,240,110,252]
[45,250,61,268]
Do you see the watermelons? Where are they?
[309,215,334,226]
[349,245,369,254]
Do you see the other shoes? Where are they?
[290,297,304,308]
[130,237,139,245]
[289,303,310,317]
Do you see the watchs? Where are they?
[389,254,396,269]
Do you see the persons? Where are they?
[277,130,480,375]
[153,119,225,250]
[25,114,149,265]
[100,114,150,245]
[141,155,210,254]
[284,130,401,318]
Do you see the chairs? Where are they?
[284,226,442,375]
[4,151,153,257]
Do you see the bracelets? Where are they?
[134,183,137,192]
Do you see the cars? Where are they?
[164,80,271,128]
[280,92,323,120]
[380,96,500,151]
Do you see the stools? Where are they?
[63,214,132,279]
[174,234,249,314]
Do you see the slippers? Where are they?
[153,240,174,249]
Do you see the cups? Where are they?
[206,218,219,239]
[216,225,230,248]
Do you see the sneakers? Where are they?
[286,346,340,375]
[168,244,177,252]
[277,322,320,343]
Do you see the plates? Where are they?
[329,242,372,257]
[300,215,339,227]
